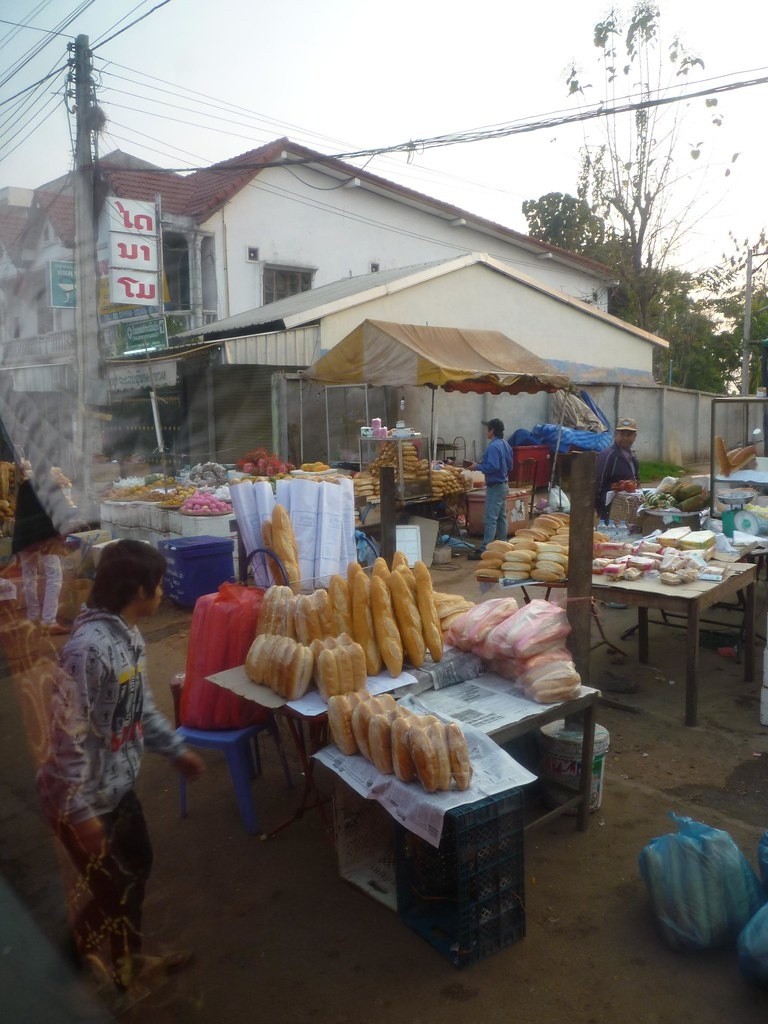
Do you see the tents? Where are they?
[299,318,571,514]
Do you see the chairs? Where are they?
[515,458,538,519]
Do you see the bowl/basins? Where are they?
[717,493,755,504]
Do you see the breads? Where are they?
[473,512,704,584]
[262,505,301,593]
[282,440,470,526]
[640,831,760,951]
[716,436,756,477]
[243,550,582,794]
[0,459,83,538]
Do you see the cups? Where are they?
[755,457,768,471]
[372,419,387,438]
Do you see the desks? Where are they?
[272,636,603,836]
[398,491,469,546]
[476,555,756,727]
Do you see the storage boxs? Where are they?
[357,434,430,501]
[157,534,235,607]
[330,768,528,972]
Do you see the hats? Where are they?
[615,417,637,432]
[481,418,504,433]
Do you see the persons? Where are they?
[38,539,204,991]
[13,457,69,634]
[595,419,642,607]
[468,419,513,559]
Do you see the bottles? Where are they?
[462,470,485,488]
[618,520,628,542]
[597,519,606,534]
[607,518,617,542]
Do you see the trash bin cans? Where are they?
[510,441,555,488]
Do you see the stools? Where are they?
[175,713,295,838]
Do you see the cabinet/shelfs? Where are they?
[709,396,768,532]
[436,436,466,468]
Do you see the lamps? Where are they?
[400,396,405,410]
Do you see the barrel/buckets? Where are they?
[540,720,610,810]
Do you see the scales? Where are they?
[716,491,759,539]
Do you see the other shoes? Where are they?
[468,549,483,560]
[46,624,69,634]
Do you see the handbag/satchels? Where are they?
[638,811,767,982]
